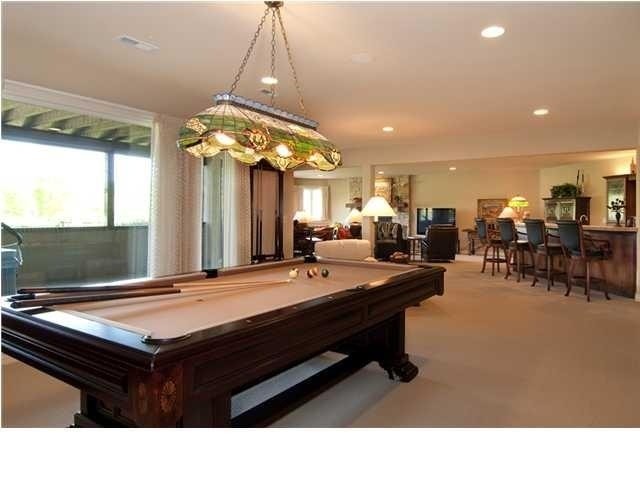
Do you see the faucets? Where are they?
[580,215,588,225]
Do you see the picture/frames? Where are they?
[476,198,508,223]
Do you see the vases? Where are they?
[614,210,623,226]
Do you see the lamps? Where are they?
[508,193,529,217]
[175,2,345,172]
[359,195,397,264]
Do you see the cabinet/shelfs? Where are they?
[607,178,635,222]
[541,198,593,224]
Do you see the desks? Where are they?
[1,252,447,428]
[408,233,426,263]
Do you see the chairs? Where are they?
[461,217,613,303]
[294,209,362,255]
[2,221,26,295]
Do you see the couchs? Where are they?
[420,223,459,262]
[375,221,411,259]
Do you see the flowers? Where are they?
[606,197,626,213]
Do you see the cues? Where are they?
[257,163,262,256]
[11,279,290,308]
[275,175,281,261]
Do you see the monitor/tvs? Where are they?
[415,207,456,236]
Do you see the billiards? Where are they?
[289,267,328,278]
